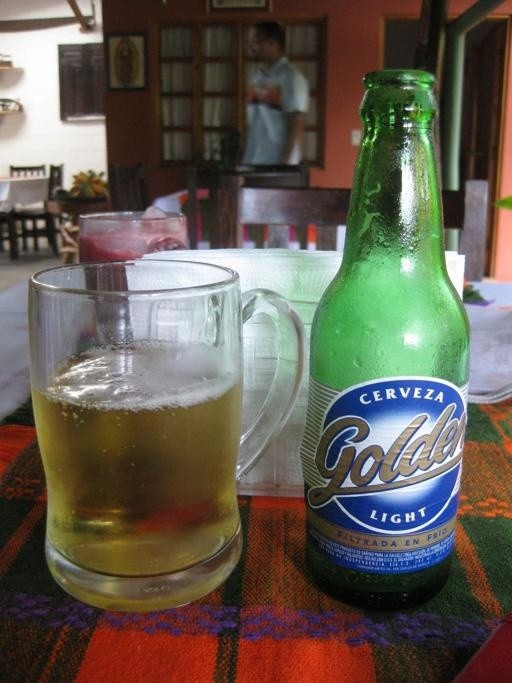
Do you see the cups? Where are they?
[76,209,191,264]
[27,254,306,614]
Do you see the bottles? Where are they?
[304,68,471,614]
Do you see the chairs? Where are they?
[108,158,490,283]
[6,165,63,258]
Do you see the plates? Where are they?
[463,282,511,406]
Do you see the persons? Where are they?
[240,21,309,172]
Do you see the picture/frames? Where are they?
[105,33,148,92]
[205,2,274,15]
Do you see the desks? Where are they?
[0,247,512,682]
[48,191,112,266]
[1,176,51,259]
